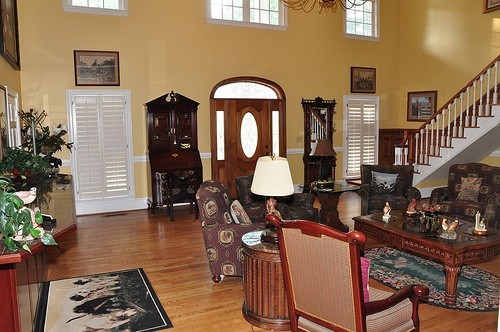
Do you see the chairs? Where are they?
[267,215,430,332]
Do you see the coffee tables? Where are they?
[352,212,500,305]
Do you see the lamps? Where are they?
[310,139,337,188]
[281,0,368,14]
[250,151,294,243]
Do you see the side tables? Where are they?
[299,183,360,231]
[242,231,292,332]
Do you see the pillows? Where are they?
[360,257,371,303]
[456,177,482,202]
[371,171,399,194]
[230,200,252,225]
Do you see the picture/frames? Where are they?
[73,50,120,86]
[407,90,438,122]
[351,66,376,93]
[0,0,21,71]
[482,0,500,13]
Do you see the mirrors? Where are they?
[301,97,337,153]
[0,85,6,160]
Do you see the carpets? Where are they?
[34,268,174,332]
[362,246,500,311]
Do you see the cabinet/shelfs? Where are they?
[0,248,49,332]
[142,91,203,221]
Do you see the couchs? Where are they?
[196,175,319,283]
[361,164,500,232]
